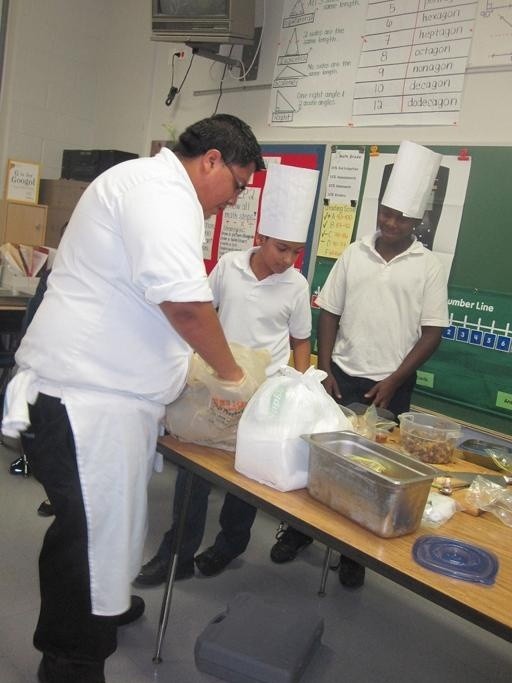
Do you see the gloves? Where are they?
[0,367,38,437]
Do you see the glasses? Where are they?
[223,155,247,195]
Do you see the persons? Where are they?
[131,162,320,588]
[14,114,267,683]
[271,140,449,587]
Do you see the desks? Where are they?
[152,426,512,665]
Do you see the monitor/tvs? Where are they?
[151,0,256,46]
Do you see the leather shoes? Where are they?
[11,457,32,473]
[38,497,55,516]
[117,595,145,626]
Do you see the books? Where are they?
[0,242,56,278]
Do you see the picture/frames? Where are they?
[5,158,41,204]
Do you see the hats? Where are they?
[381,139,443,219]
[257,162,320,243]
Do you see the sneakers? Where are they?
[194,546,226,576]
[340,555,365,588]
[271,525,313,563]
[136,557,195,585]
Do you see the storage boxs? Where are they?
[194,601,325,681]
[61,149,139,183]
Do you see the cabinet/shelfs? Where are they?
[2,201,48,246]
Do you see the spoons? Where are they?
[438,484,471,495]
